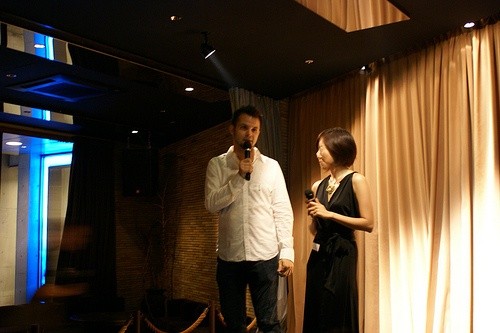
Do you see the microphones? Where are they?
[304,189,324,231]
[243,141,251,181]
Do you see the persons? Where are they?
[204,104,295,333]
[302,128,375,333]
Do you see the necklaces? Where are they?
[326,168,346,194]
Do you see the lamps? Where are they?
[199,42,217,60]
